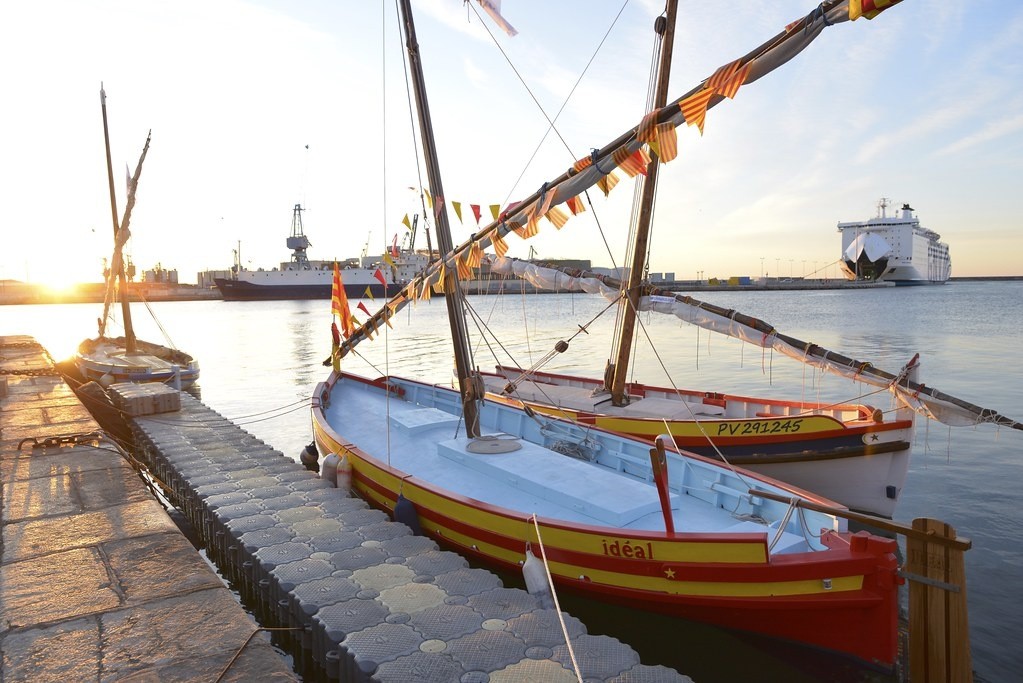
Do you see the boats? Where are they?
[834,196,952,285]
[212,202,429,300]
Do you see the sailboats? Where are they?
[72,80,202,390]
[310,0,905,669]
[470,0,925,466]
